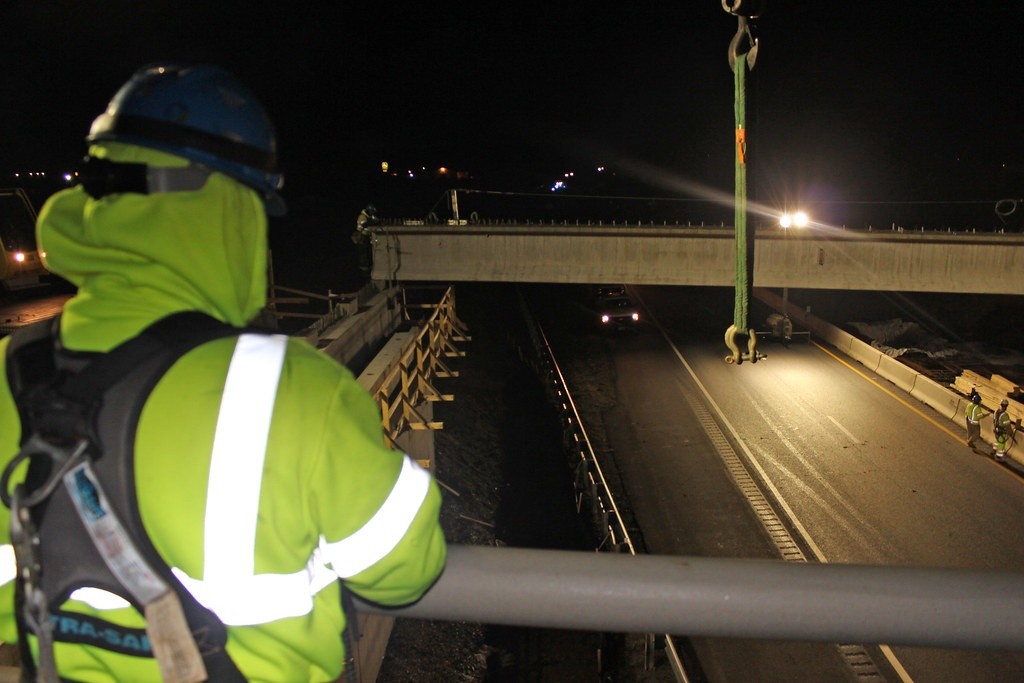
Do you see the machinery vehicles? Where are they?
[1,184,57,296]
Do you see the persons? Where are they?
[1,61,449,683]
[990,400,1018,460]
[964,395,990,448]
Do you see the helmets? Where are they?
[81,64,283,197]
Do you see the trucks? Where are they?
[592,283,639,334]
